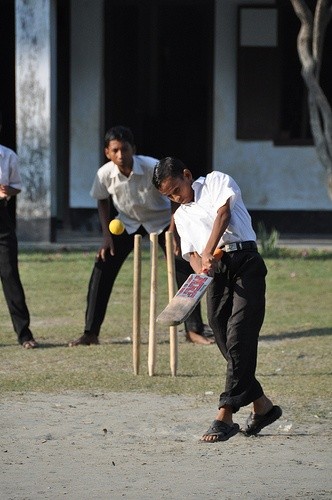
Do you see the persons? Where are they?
[65,127,216,347]
[153,157,282,442]
[0,144,39,348]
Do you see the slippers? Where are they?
[243,405,282,437]
[199,420,239,443]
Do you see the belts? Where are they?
[222,241,257,253]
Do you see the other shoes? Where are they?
[22,340,38,349]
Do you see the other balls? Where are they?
[108,218,124,235]
